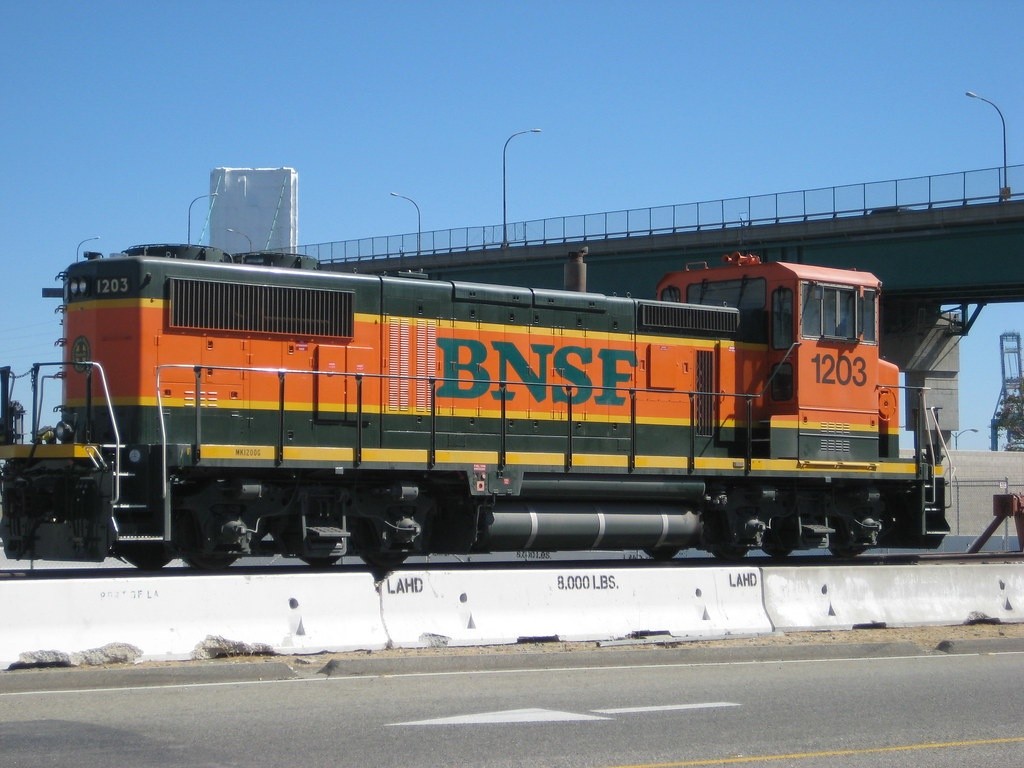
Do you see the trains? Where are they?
[0,241,964,579]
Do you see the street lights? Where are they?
[950,427,979,450]
[187,192,219,246]
[76,235,101,262]
[501,126,541,247]
[390,191,421,258]
[965,87,1010,203]
[227,228,254,253]
